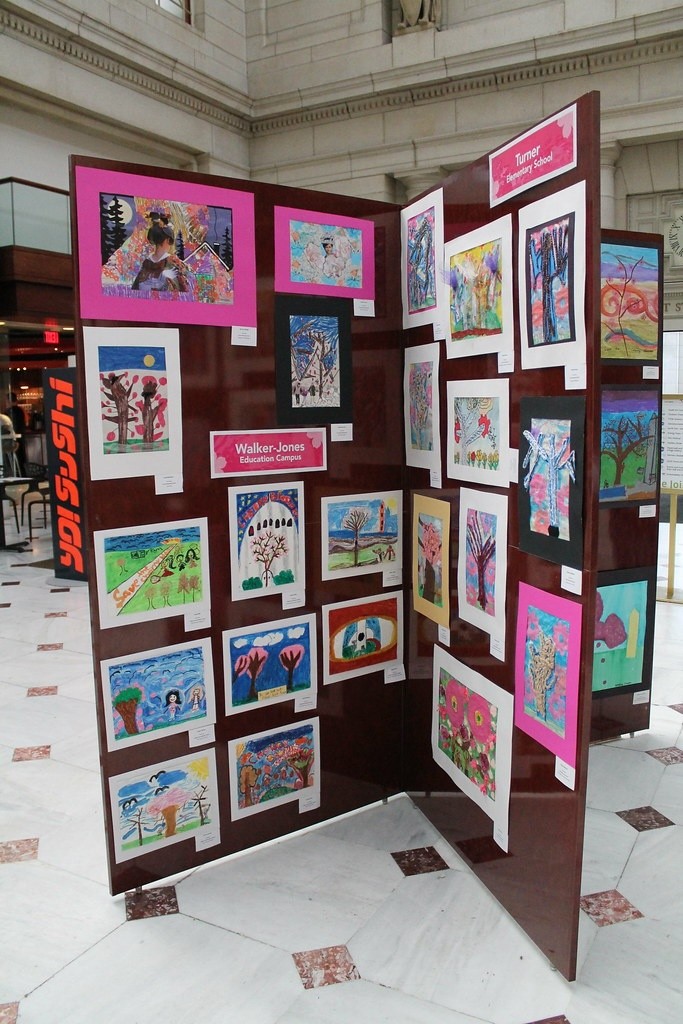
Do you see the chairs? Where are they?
[0,462,52,542]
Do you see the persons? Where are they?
[0,392,28,477]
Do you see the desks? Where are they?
[0,476,46,553]
